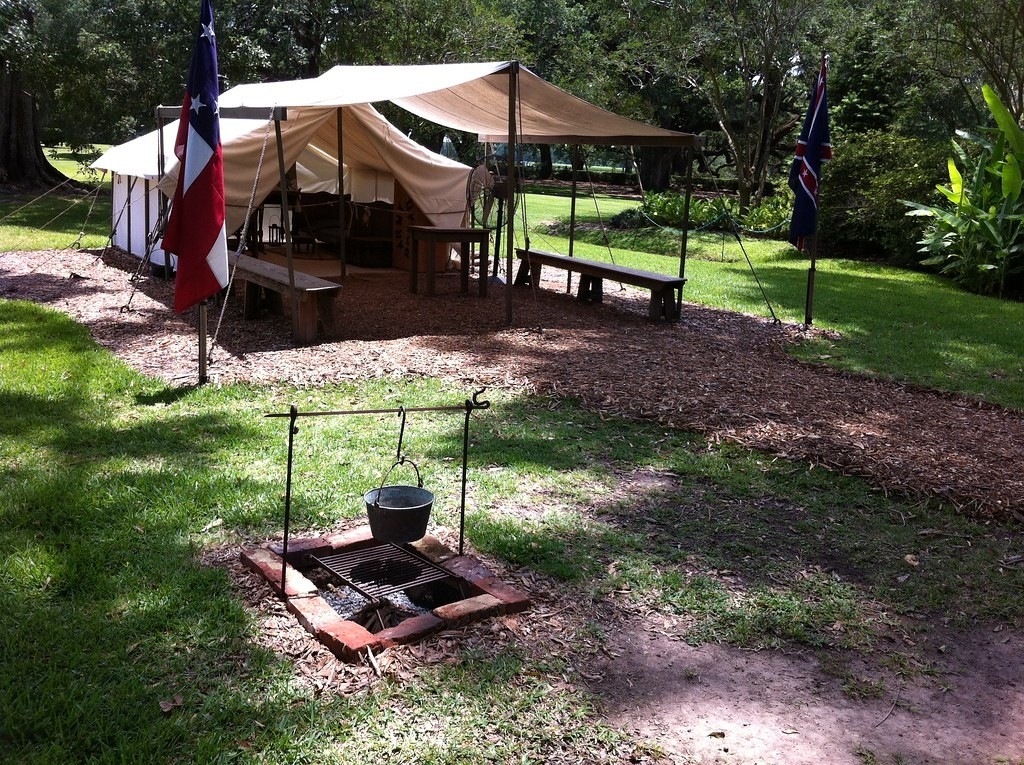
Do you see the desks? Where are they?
[408,225,492,298]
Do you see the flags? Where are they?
[160,0,228,313]
[788,57,832,253]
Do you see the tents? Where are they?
[91,61,695,343]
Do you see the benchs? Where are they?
[151,250,342,346]
[514,247,688,326]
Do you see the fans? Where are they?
[466,153,520,285]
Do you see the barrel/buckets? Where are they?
[363,459,435,542]
